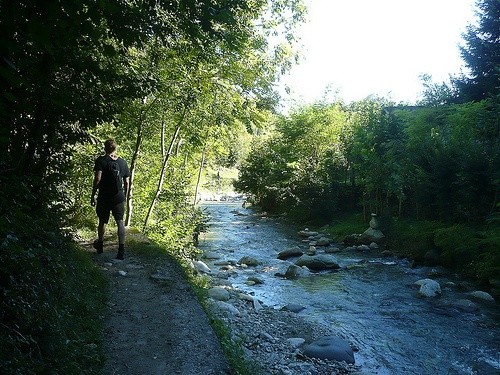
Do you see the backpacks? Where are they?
[103,156,125,204]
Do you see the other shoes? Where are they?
[93,240,103,254]
[117,248,125,260]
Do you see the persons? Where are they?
[90,139,131,260]
